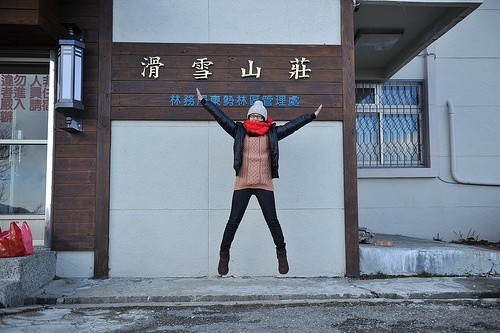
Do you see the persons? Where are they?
[196,88,322,275]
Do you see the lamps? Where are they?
[55,21,88,135]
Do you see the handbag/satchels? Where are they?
[0,220,33,258]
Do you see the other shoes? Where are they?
[217,257,229,275]
[278,255,289,274]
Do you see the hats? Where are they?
[247,101,267,121]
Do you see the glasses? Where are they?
[249,115,263,119]
